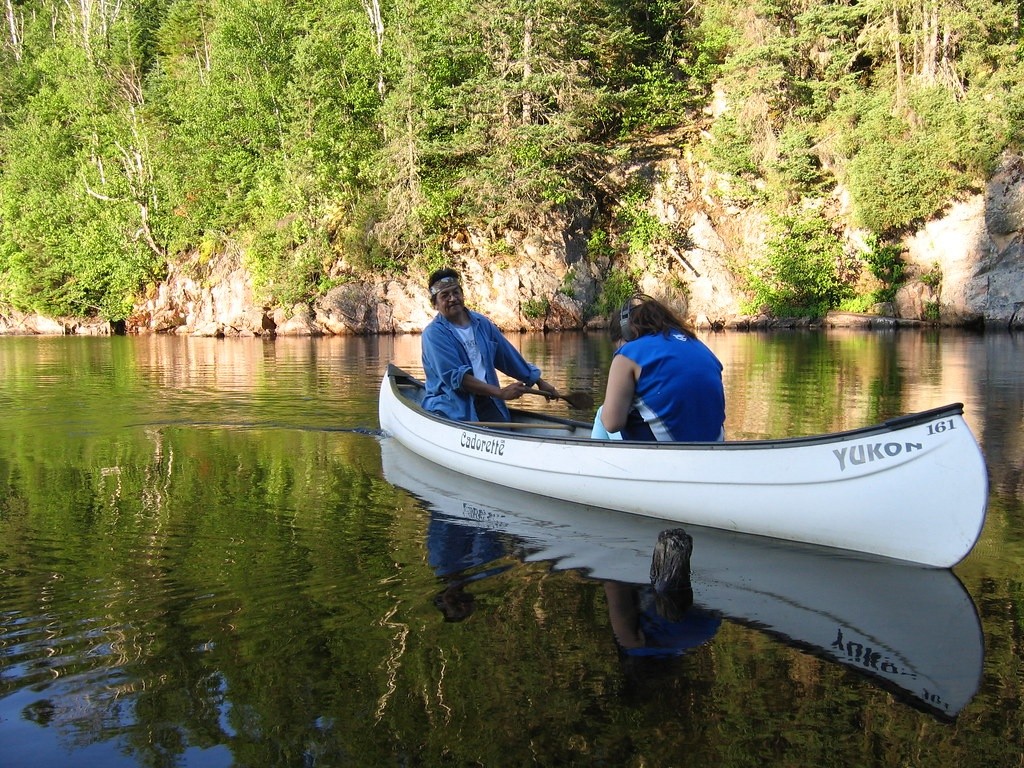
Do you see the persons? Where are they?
[605,579,722,709]
[415,269,562,434]
[425,509,506,621]
[591,299,724,444]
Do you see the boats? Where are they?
[379,434,987,725]
[378,364,989,571]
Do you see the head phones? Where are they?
[619,294,655,341]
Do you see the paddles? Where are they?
[524,386,595,410]
